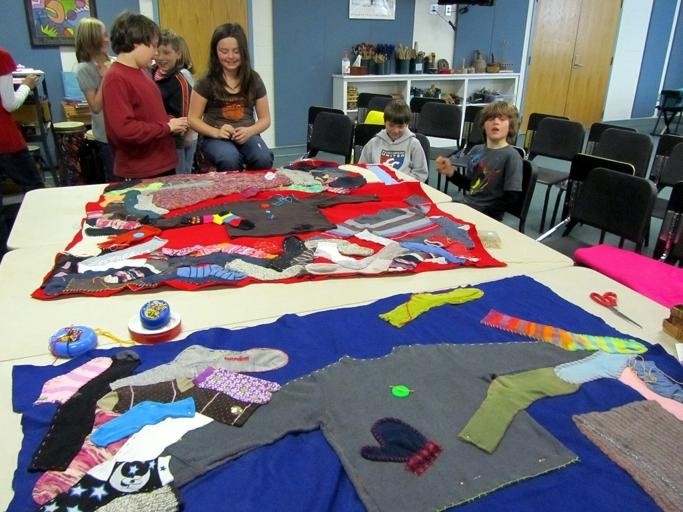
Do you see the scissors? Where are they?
[590,292,643,329]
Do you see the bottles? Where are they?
[341,51,350,76]
[414,41,418,57]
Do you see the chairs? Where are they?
[302,93,430,186]
[430,89,682,268]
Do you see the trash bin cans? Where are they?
[82,129,105,183]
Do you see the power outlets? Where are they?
[429,4,439,15]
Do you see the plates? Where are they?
[51,121,84,131]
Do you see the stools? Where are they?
[82,129,98,165]
[48,119,85,164]
[24,145,51,179]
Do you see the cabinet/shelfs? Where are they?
[330,71,520,156]
[10,73,63,187]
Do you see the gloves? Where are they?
[361,416,444,479]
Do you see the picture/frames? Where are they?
[23,0,99,50]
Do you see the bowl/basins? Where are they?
[350,67,368,75]
[486,67,499,73]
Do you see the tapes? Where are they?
[127,314,182,345]
[438,59,450,68]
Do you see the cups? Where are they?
[360,59,423,74]
[427,68,437,74]
[474,60,485,73]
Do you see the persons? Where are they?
[0,46,44,261]
[103,10,188,181]
[190,23,277,172]
[148,30,190,176]
[359,97,427,185]
[74,17,119,183]
[434,100,522,224]
[178,37,198,175]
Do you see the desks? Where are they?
[61,99,93,128]
[5,163,452,251]
[0,265,682,511]
[0,201,575,361]
